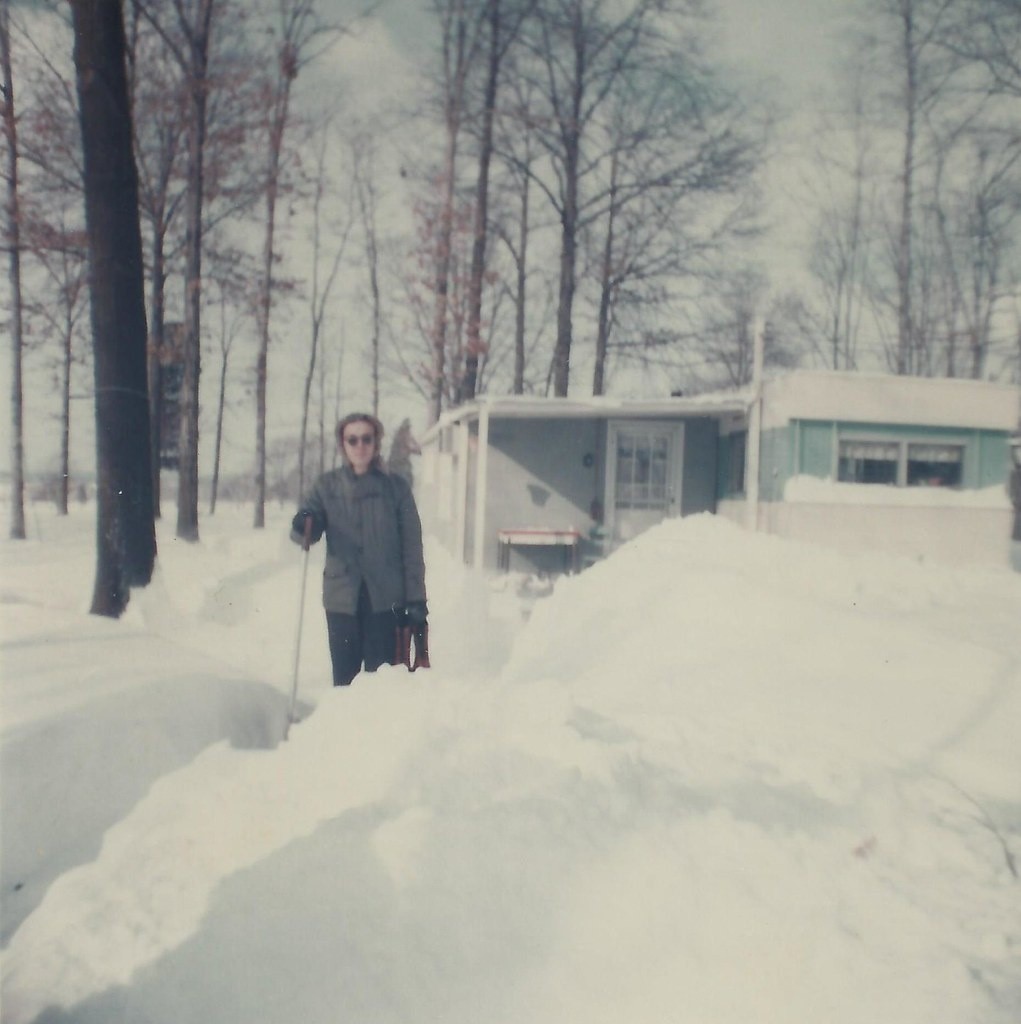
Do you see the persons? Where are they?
[290,413,430,686]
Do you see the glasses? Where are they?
[344,436,372,446]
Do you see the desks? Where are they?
[497,529,580,576]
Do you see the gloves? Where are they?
[292,509,326,536]
[407,606,429,622]
